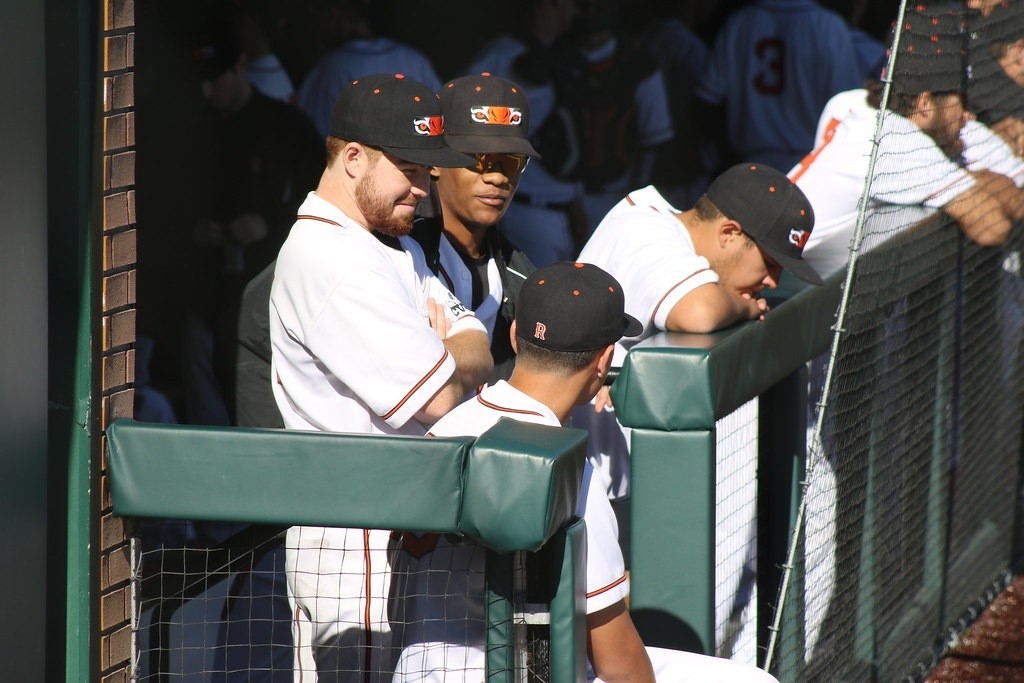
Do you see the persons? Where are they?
[575,162,826,566]
[292,0,441,141]
[565,0,678,239]
[683,0,860,176]
[165,32,327,429]
[396,76,549,379]
[265,72,495,683]
[784,0,1024,382]
[387,262,782,683]
[633,0,712,79]
[467,0,581,269]
[240,17,291,103]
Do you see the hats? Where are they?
[706,162,824,286]
[516,263,644,352]
[440,72,542,159]
[331,73,480,168]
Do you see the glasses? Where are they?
[475,153,530,173]
